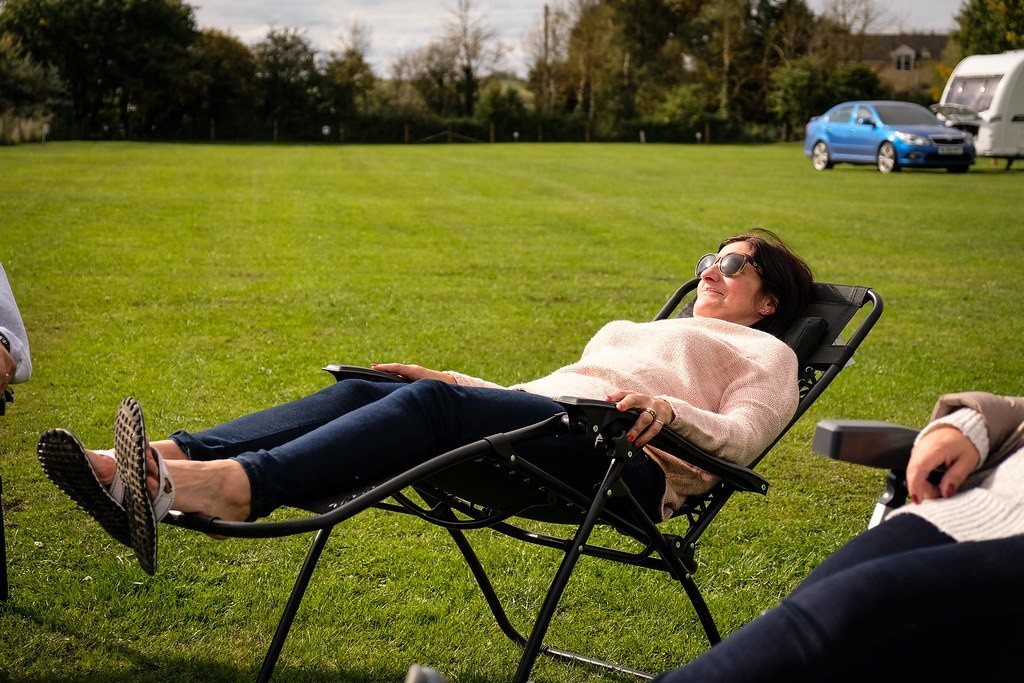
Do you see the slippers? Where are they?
[38,428,134,548]
[114,396,174,576]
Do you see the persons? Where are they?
[0,263,32,602]
[404,393,1024,683]
[36,228,818,574]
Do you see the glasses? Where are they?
[695,252,765,278]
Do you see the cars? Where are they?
[803,101,976,173]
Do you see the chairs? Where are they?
[156,277,884,683]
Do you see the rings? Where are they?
[6,373,9,377]
[656,420,663,426]
[644,408,655,417]
